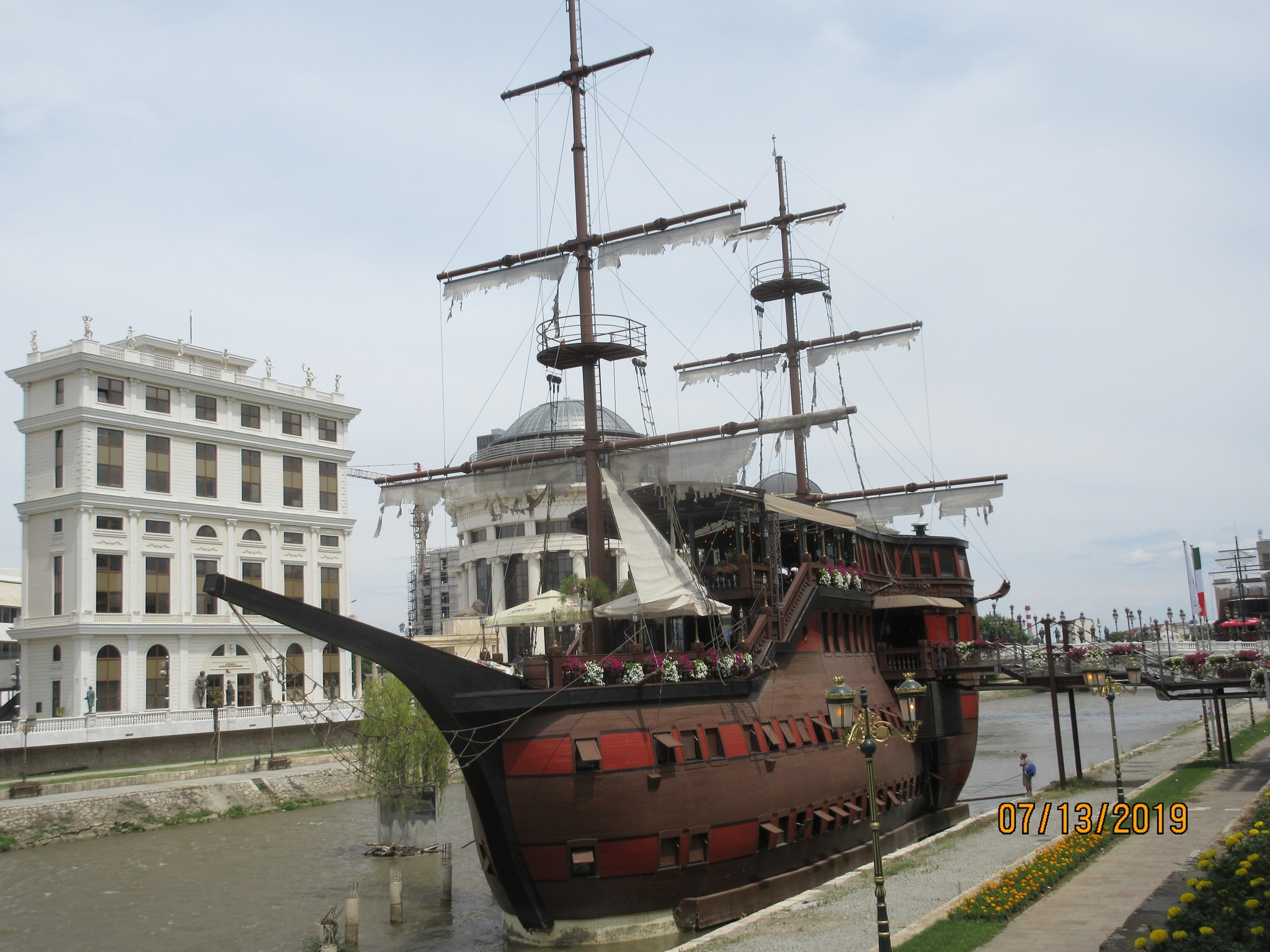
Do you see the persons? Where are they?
[195,671,209,708]
[512,650,530,662]
[85,686,96,714]
[1039,627,1046,644]
[1104,626,1110,641]
[330,676,336,698]
[226,680,234,705]
[1142,623,1150,641]
[1020,752,1034,797]
[261,670,274,703]
[668,642,680,652]
[1053,627,1061,642]
[1090,625,1096,642]
[1077,626,1084,644]
[643,643,654,653]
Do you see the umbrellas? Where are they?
[538,589,539,591]
[1219,618,1265,627]
[591,589,733,653]
[485,591,594,625]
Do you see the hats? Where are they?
[1021,753,1027,757]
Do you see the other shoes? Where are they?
[1024,794,1033,797]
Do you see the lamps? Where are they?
[495,621,500,653]
[762,574,769,607]
[763,528,770,558]
[803,524,808,554]
[479,614,487,651]
[836,533,843,560]
[694,609,700,643]
[632,610,638,644]
[739,523,746,555]
[852,535,857,563]
[820,529,826,557]
[521,623,527,655]
[739,607,746,642]
[701,551,719,565]
[551,606,558,646]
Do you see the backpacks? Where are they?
[1025,764,1036,777]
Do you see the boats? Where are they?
[194,0,1021,948]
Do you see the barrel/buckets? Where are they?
[522,655,547,689]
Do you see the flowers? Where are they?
[1028,643,1143,667]
[818,562,865,592]
[1162,648,1270,696]
[940,638,992,664]
[562,648,753,686]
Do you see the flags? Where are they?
[1183,542,1203,615]
[1191,545,1207,616]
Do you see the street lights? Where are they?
[991,601,1186,686]
[1080,653,1142,822]
